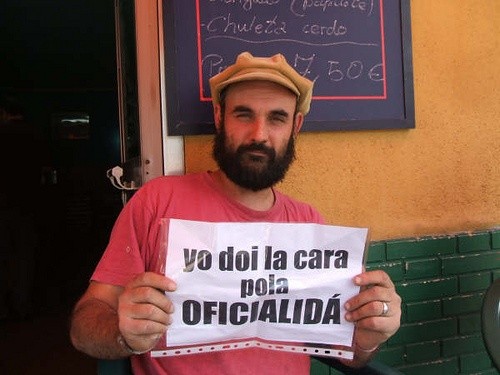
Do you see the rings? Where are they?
[383,301,388,314]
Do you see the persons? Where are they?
[0,96,42,325]
[70,52,402,375]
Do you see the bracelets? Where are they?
[117,334,158,355]
[356,346,377,352]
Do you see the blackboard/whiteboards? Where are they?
[162,0,416,136]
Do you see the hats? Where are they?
[208,52,313,116]
